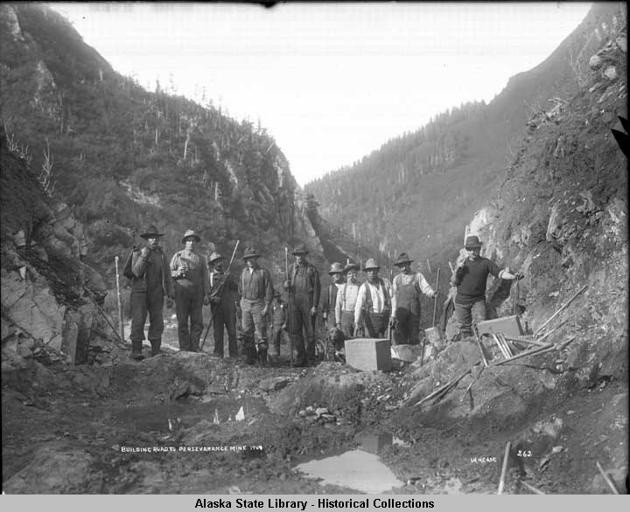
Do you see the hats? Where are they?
[208,252,225,264]
[243,247,261,258]
[140,227,164,237]
[363,258,380,271]
[394,253,413,265]
[182,230,200,243]
[292,247,308,255]
[466,236,481,248]
[328,262,356,274]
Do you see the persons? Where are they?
[354,258,397,339]
[270,290,294,365]
[334,263,360,339]
[392,253,440,344]
[281,244,321,367]
[208,252,240,362]
[322,262,346,334]
[170,229,210,352]
[238,248,273,369]
[451,237,520,339]
[129,224,172,360]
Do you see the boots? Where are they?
[129,341,144,359]
[150,339,162,357]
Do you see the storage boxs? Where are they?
[476,315,525,337]
[344,337,393,372]
